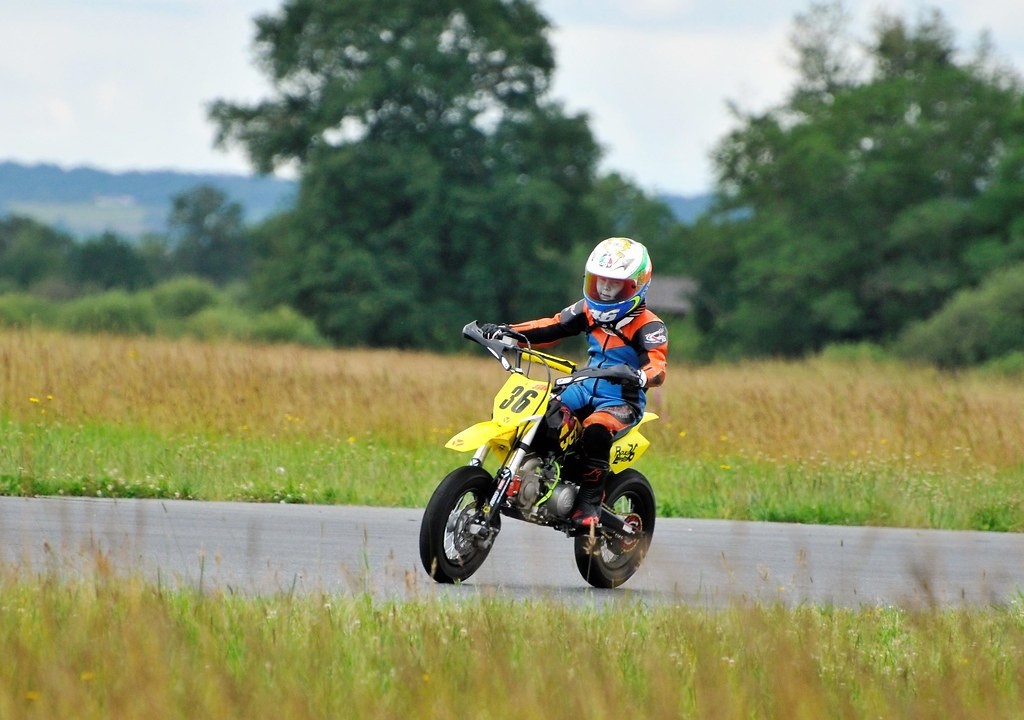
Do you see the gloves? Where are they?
[482,323,511,339]
[607,368,647,388]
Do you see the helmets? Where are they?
[583,238,652,322]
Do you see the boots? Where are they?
[571,460,610,527]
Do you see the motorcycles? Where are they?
[419,320,660,589]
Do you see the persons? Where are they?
[479,237,668,551]
